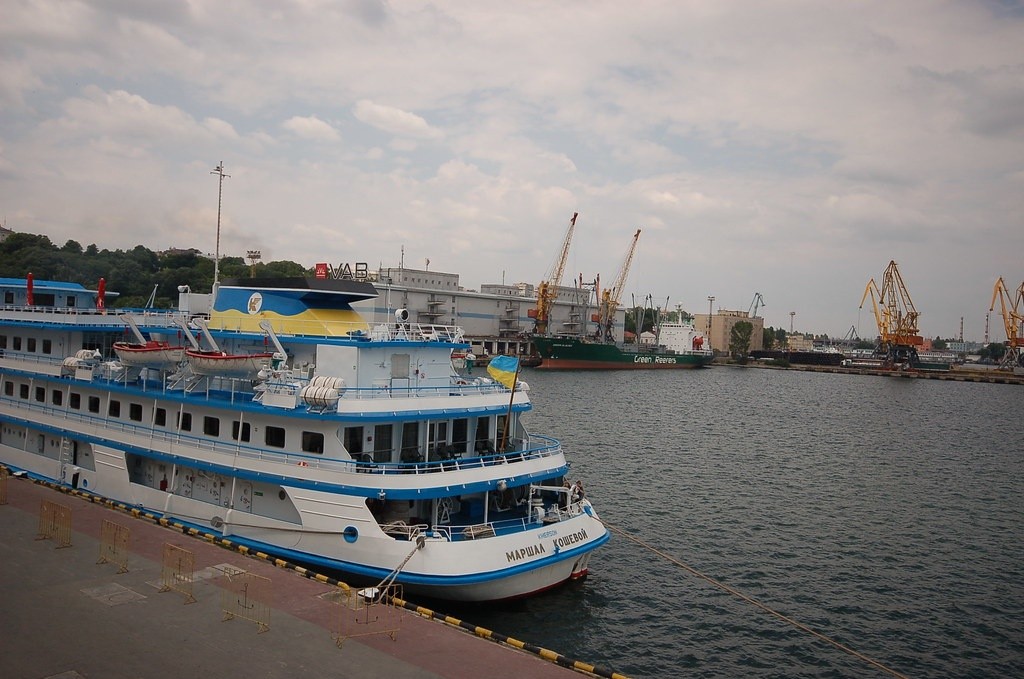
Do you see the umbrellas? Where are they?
[27,272,33,305]
[97,278,105,308]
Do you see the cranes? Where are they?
[594,230,641,344]
[530,211,579,333]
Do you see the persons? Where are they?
[562,480,585,504]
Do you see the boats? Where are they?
[529,335,717,370]
[188,347,273,375]
[0,272,611,613]
[112,340,185,370]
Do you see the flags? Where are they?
[486,355,518,390]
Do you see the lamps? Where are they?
[377,492,386,500]
[178,284,192,294]
[258,364,273,381]
